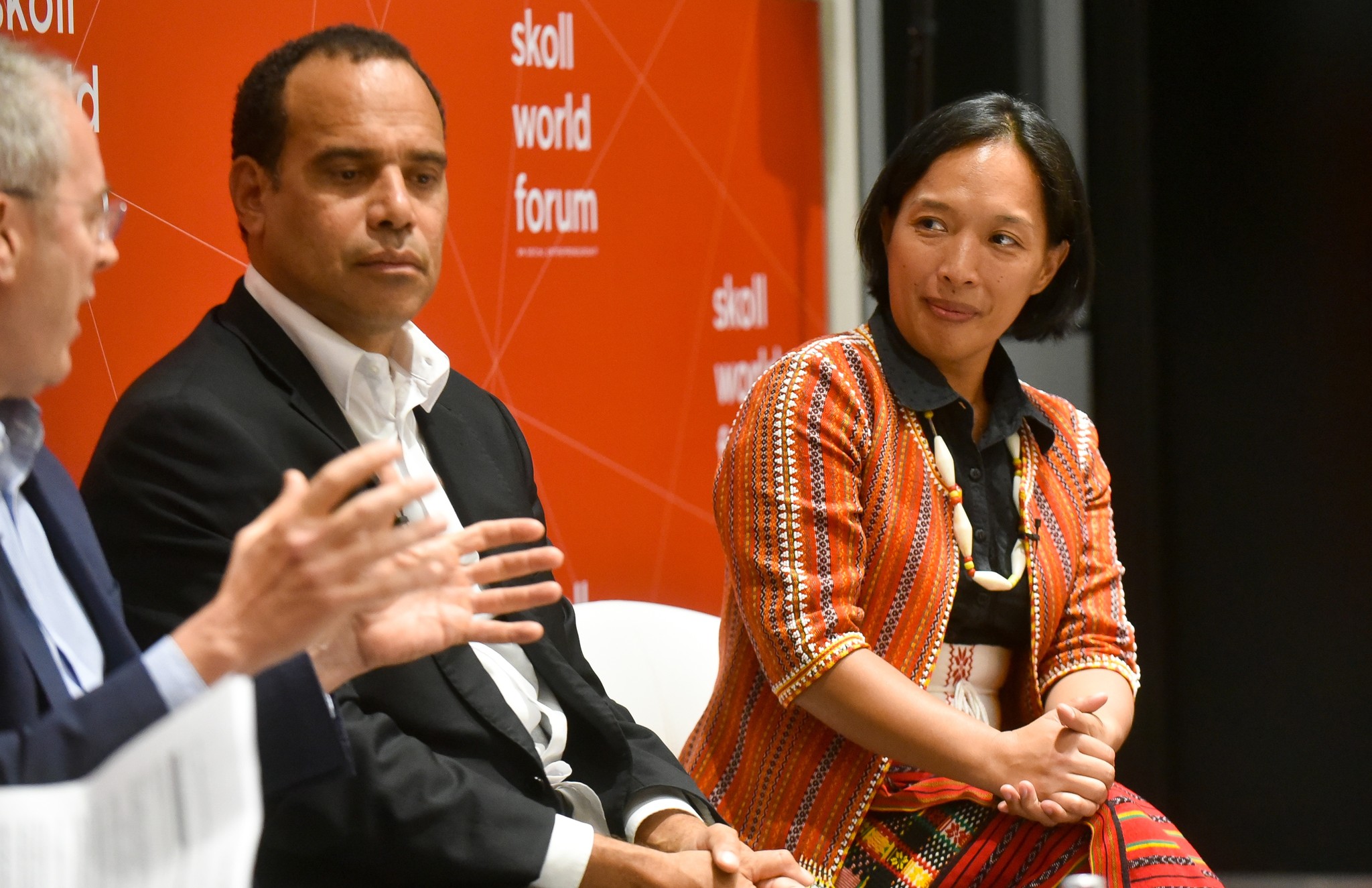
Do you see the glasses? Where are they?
[0,187,125,244]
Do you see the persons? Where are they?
[78,19,815,888]
[675,89,1227,888]
[0,41,565,888]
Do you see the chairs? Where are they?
[571,594,724,753]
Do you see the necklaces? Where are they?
[918,406,1033,592]
